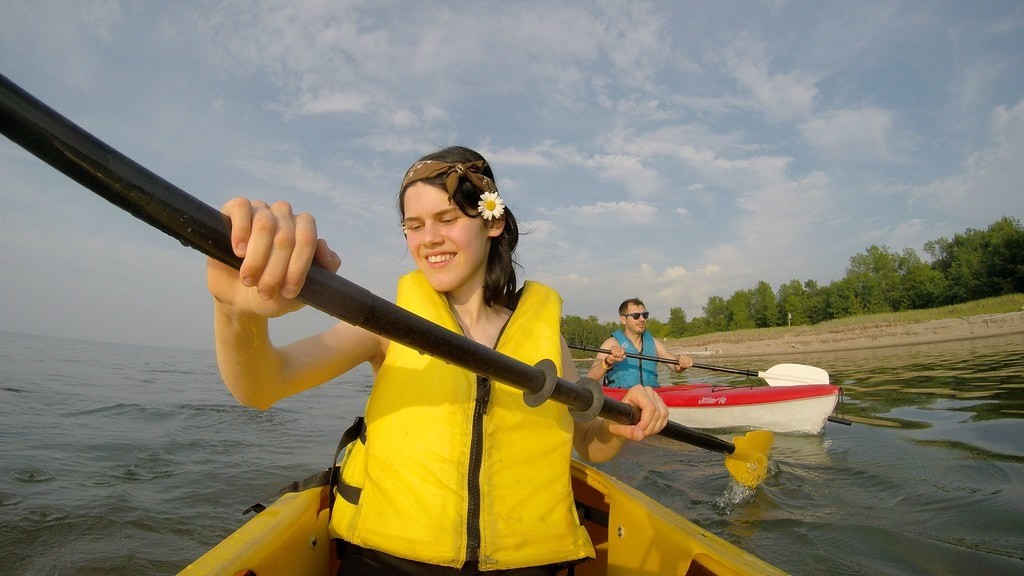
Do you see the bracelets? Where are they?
[602,360,613,369]
[605,357,614,366]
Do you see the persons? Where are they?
[206,146,669,576]
[584,299,693,389]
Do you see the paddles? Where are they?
[568,345,830,387]
[0,73,777,487]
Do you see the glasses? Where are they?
[623,312,649,320]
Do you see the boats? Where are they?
[173,453,789,576]
[602,385,844,436]
